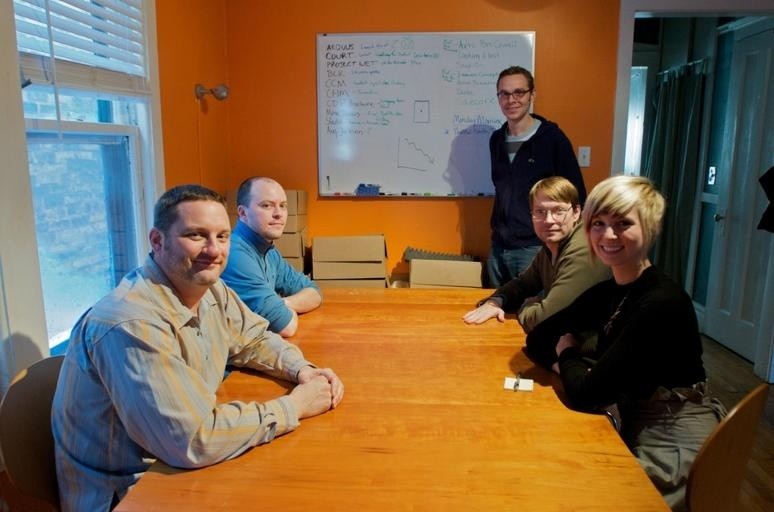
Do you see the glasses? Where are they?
[530,205,573,223]
[496,88,531,99]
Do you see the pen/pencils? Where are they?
[514,372,522,392]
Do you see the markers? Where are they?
[379,193,497,196]
[334,193,353,196]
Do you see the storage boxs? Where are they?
[223,189,484,288]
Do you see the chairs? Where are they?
[0,354,68,511]
[671,382,770,511]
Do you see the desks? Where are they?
[111,288,673,511]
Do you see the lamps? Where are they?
[194,84,229,101]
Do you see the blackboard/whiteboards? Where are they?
[317,32,536,197]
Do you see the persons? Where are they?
[484,64,588,304]
[523,173,733,510]
[460,176,613,369]
[48,184,346,511]
[209,174,324,382]
